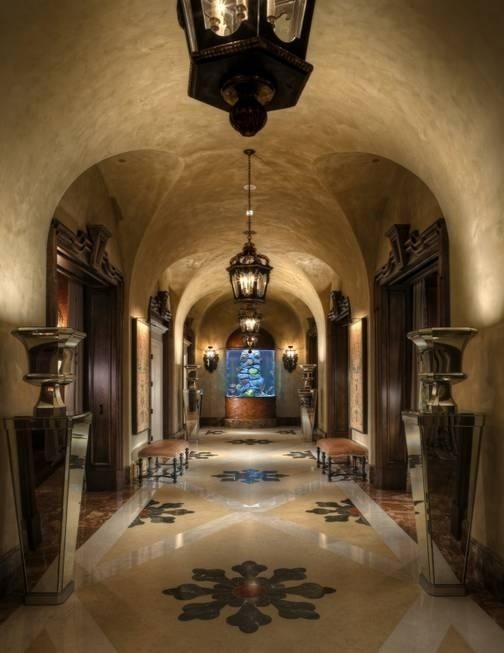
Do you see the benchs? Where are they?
[138,439,190,484]
[316,437,370,481]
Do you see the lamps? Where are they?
[233,311,263,357]
[175,1,318,139]
[225,146,274,309]
[202,343,220,374]
[278,342,302,372]
